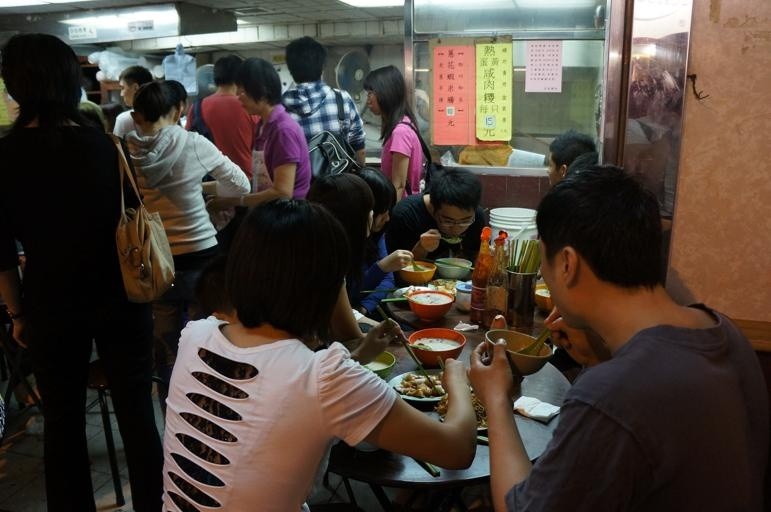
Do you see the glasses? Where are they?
[437,210,475,228]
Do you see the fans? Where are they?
[194,64,218,99]
[334,49,372,103]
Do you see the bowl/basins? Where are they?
[486,328,555,376]
[398,256,473,323]
[409,327,467,367]
[365,350,397,379]
[536,281,552,313]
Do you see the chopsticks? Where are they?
[358,288,408,303]
[423,461,441,476]
[376,304,441,396]
[507,238,543,272]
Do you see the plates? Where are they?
[439,416,488,432]
[489,207,538,231]
[389,369,446,404]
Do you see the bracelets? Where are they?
[4,309,21,321]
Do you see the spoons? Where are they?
[517,327,553,356]
[441,236,463,244]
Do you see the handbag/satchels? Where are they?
[305,130,363,180]
[115,204,176,305]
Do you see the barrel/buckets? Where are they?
[488,208,539,267]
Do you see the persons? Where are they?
[561,151,599,173]
[2,32,422,424]
[0,33,166,512]
[387,165,486,280]
[303,172,375,344]
[469,163,771,511]
[546,130,597,188]
[352,165,415,333]
[157,196,480,512]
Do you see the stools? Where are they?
[70,368,155,509]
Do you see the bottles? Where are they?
[485,229,509,326]
[471,227,493,324]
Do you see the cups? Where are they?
[506,264,539,335]
[87,51,166,84]
